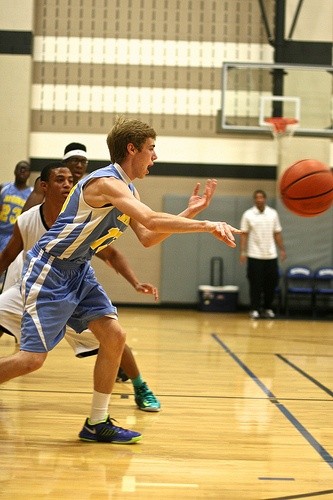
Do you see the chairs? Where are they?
[260,266,333,319]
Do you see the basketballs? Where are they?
[281,159,333,218]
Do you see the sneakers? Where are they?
[117,368,129,382]
[134,382,160,411]
[79,415,142,443]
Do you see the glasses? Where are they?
[68,158,88,167]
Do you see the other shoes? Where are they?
[260,309,274,317]
[249,310,259,318]
